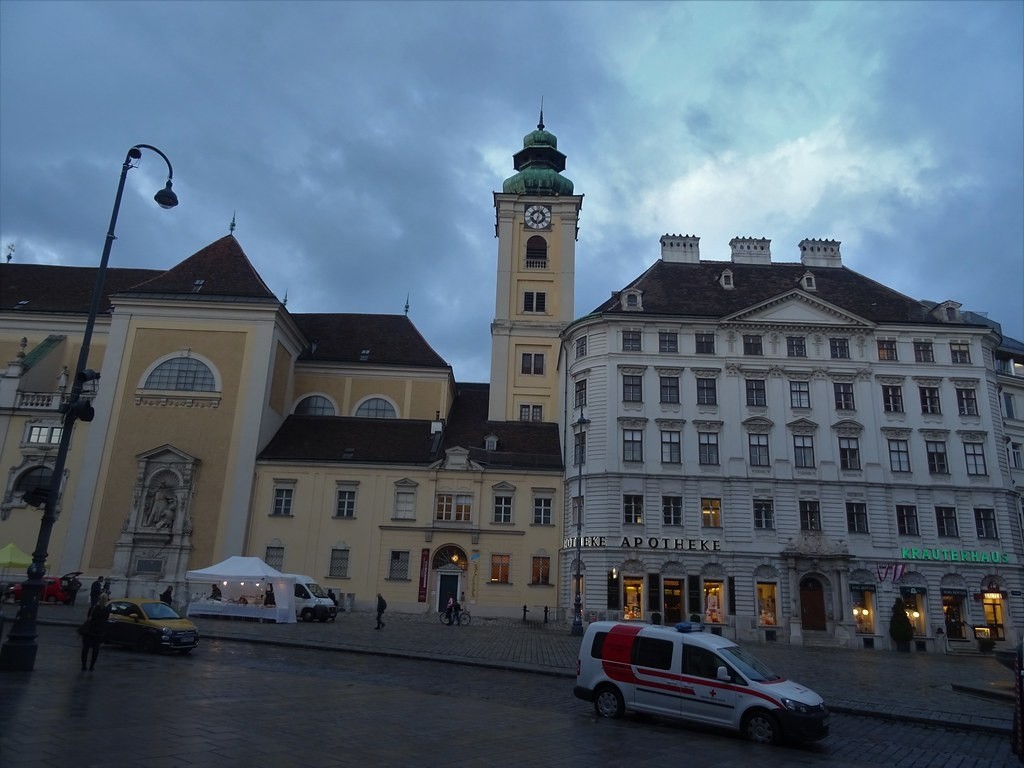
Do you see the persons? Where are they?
[143,481,178,527]
[327,589,336,622]
[207,584,221,601]
[86,576,104,618]
[162,586,173,605]
[374,593,387,630]
[445,592,461,625]
[68,576,82,607]
[81,592,112,672]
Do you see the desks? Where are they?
[186,600,288,623]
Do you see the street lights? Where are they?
[571,402,591,637]
[0,144,181,667]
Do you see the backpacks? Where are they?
[381,599,386,609]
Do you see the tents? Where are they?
[184,556,298,623]
[0,543,51,604]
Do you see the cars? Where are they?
[102,598,201,656]
[9,571,83,605]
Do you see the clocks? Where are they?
[524,204,552,230]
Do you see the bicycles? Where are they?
[439,609,471,626]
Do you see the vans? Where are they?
[285,573,336,622]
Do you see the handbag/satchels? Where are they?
[77,622,89,636]
[334,601,339,606]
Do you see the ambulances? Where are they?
[574,620,830,744]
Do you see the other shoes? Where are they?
[89,665,95,672]
[82,664,87,669]
[375,627,380,630]
[381,623,385,626]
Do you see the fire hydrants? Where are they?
[544,605,548,623]
[522,604,527,621]
